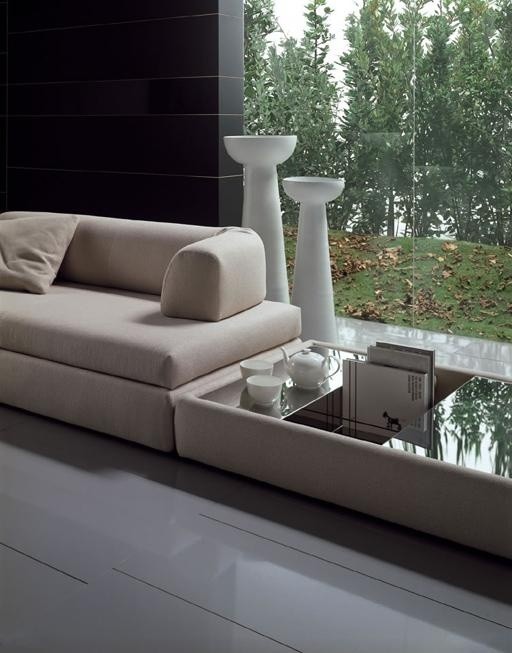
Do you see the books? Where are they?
[340,340,434,448]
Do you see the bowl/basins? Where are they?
[239,358,274,388]
[245,374,284,407]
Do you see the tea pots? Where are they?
[280,345,342,391]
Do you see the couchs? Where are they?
[0,206,300,457]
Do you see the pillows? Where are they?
[0,210,84,294]
